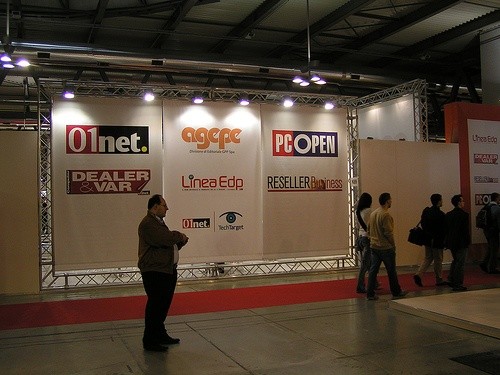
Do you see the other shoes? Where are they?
[144,344,168,351]
[436,280,446,286]
[414,274,423,287]
[367,295,378,301]
[446,280,467,291]
[488,269,500,274]
[161,333,180,344]
[356,288,368,293]
[376,287,384,290]
[392,292,407,299]
[480,263,488,273]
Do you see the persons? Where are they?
[356,192,384,293]
[480,192,500,276]
[137,194,189,353]
[414,194,448,287]
[367,193,409,300]
[438,194,471,289]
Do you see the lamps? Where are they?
[62,84,75,99]
[324,98,334,110]
[292,0,327,86]
[239,93,250,105]
[143,89,154,101]
[283,95,293,107]
[192,91,203,104]
[0,0,30,68]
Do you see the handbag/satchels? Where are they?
[408,207,429,246]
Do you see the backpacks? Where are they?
[476,201,496,229]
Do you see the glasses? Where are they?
[161,204,166,208]
